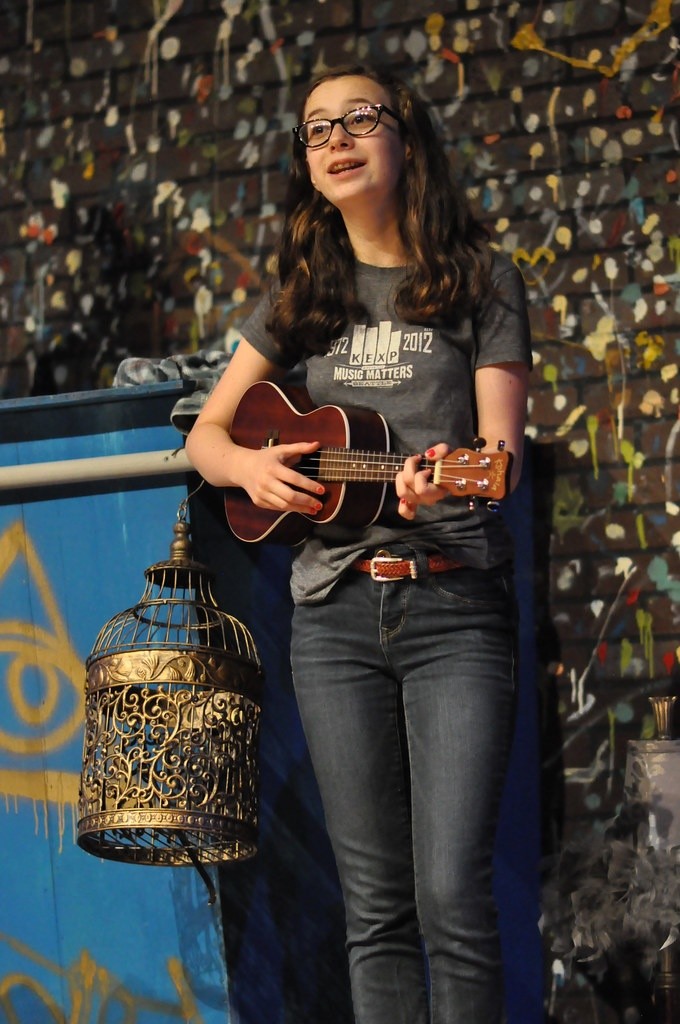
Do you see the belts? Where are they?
[355,551,469,580]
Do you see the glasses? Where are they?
[291,104,404,148]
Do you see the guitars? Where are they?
[222,382,519,546]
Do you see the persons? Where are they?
[184,64,533,1024]
